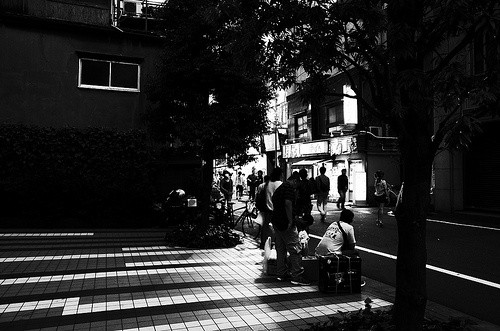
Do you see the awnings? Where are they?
[292,159,325,165]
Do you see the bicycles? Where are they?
[204,195,261,241]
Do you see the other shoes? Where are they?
[321,214,326,222]
[276,276,290,281]
[337,204,340,208]
[341,206,345,209]
[291,276,311,285]
[375,219,383,225]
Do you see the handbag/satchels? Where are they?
[262,237,277,276]
[341,232,360,256]
[295,215,315,228]
[255,189,267,211]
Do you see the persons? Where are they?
[247,169,263,200]
[257,172,282,250]
[213,170,233,218]
[314,209,367,287]
[271,174,311,286]
[315,167,330,220]
[336,169,349,210]
[374,171,390,228]
[296,168,312,235]
[235,171,247,200]
